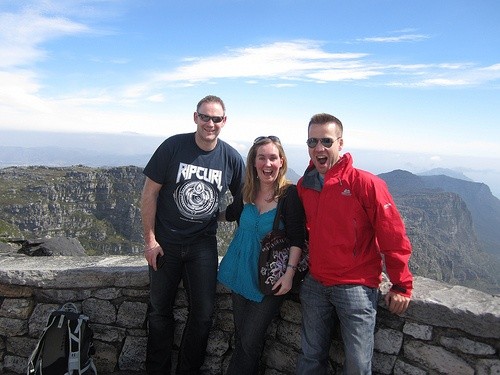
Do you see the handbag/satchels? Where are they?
[258,195,311,295]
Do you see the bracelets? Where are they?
[143,242,158,252]
[287,264,298,271]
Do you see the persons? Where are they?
[296,114,414,375]
[218,136,307,375]
[140,95,247,375]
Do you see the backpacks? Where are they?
[28,303,97,375]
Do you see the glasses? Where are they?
[252,135,281,144]
[305,137,341,148]
[197,112,225,123]
